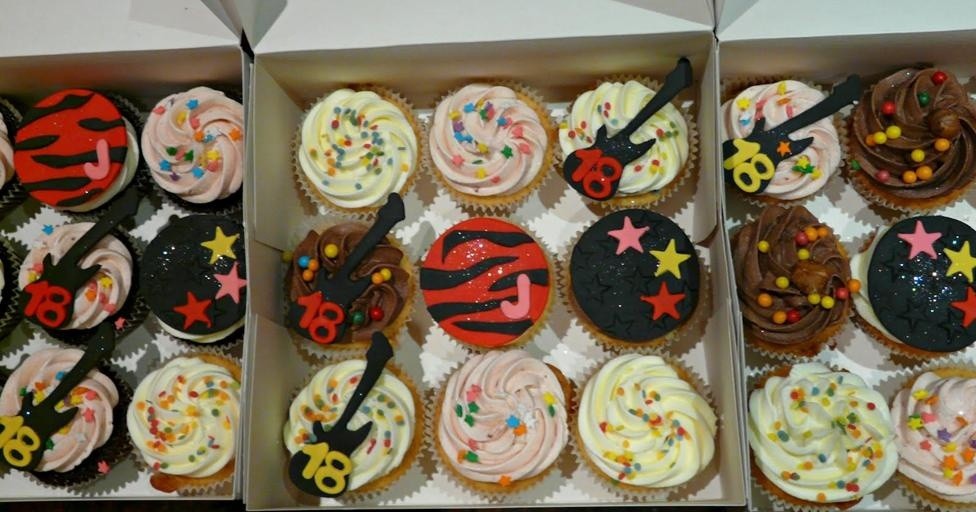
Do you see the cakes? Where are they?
[139,87,247,215]
[1,96,27,212]
[0,323,139,487]
[1,237,22,339]
[886,367,976,510]
[557,58,696,213]
[729,206,843,356]
[566,209,703,352]
[578,355,716,489]
[840,69,975,212]
[283,333,424,498]
[719,72,881,200]
[136,213,247,344]
[292,82,422,216]
[431,350,577,499]
[429,79,558,208]
[745,362,896,510]
[281,194,414,349]
[15,88,146,212]
[847,209,976,361]
[124,356,242,492]
[421,217,556,353]
[19,190,152,338]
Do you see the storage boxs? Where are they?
[0,0,248,502]
[713,0,976,511]
[243,0,748,512]
